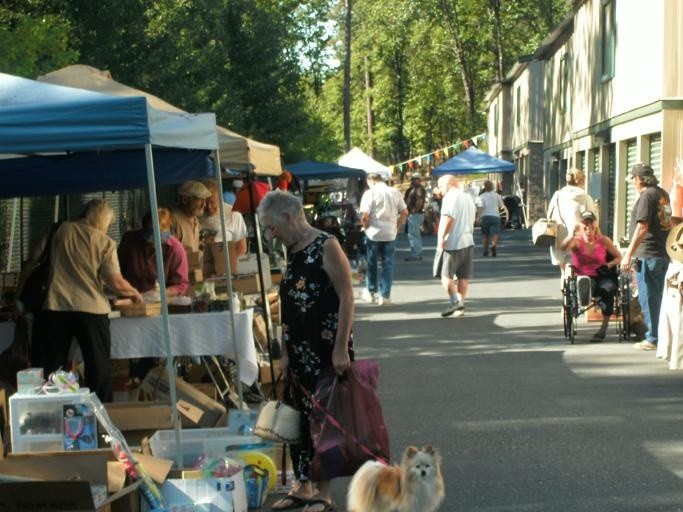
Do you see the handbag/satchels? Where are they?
[15,256,53,321]
[531,218,557,246]
[254,400,301,444]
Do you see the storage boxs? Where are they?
[113,240,279,319]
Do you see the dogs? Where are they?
[346,444,444,512]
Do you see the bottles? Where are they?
[239,291,246,311]
[233,292,239,312]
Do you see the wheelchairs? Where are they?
[560,265,630,344]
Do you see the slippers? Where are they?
[272,495,334,512]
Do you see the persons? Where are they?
[13,170,300,403]
[404,173,427,261]
[432,174,478,317]
[477,182,503,257]
[359,173,409,305]
[620,162,673,350]
[559,211,625,343]
[531,168,598,318]
[256,189,355,512]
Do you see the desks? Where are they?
[108,287,263,389]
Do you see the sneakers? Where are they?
[404,255,423,261]
[578,276,591,307]
[441,300,465,316]
[590,332,605,341]
[363,288,390,304]
[639,341,660,353]
[484,247,496,256]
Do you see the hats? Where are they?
[412,173,421,179]
[179,180,211,198]
[433,187,441,194]
[625,163,652,182]
[580,211,596,219]
[666,222,682,263]
